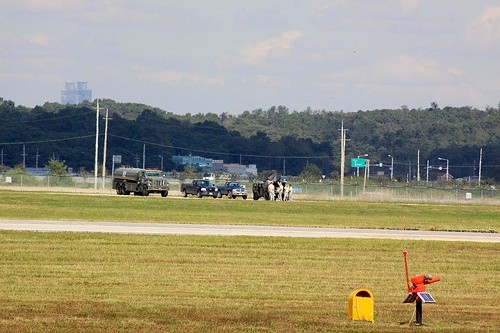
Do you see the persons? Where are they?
[284,183,289,201]
[278,181,283,201]
[289,184,293,201]
[268,182,275,201]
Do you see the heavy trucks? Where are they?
[112,168,170,197]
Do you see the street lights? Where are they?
[388,154,393,182]
[438,157,449,181]
[357,154,368,177]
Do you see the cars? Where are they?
[216,181,248,200]
[180,179,218,198]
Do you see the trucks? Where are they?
[252,180,292,201]
